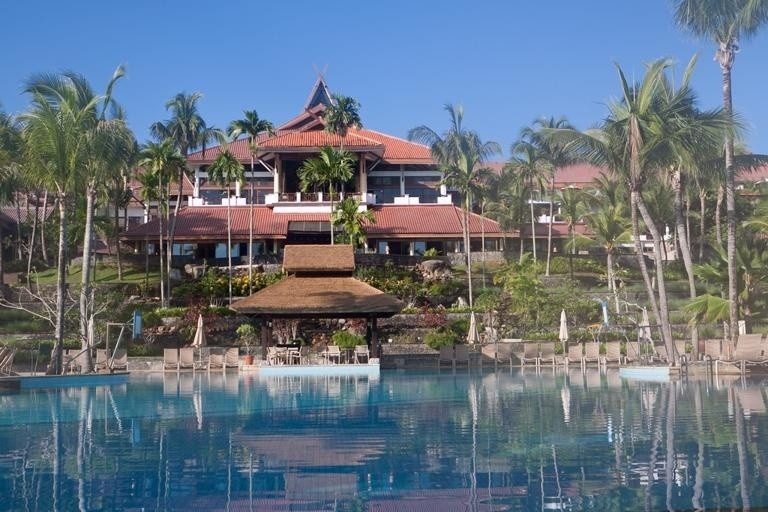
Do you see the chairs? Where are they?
[512,331,768,378]
[94,345,129,374]
[161,344,371,364]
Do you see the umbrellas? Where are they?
[465,310,481,353]
[190,315,209,368]
[467,382,480,429]
[557,307,570,356]
[192,388,205,430]
[560,380,573,426]
[638,305,655,356]
